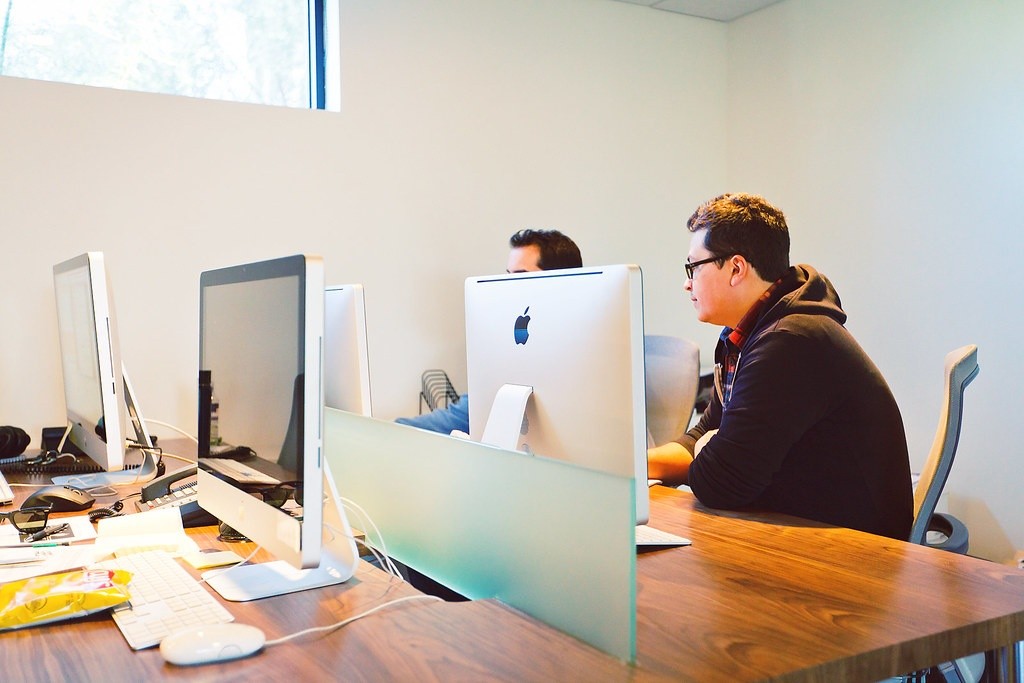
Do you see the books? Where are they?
[0,506,202,587]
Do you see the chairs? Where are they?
[644,335,700,448]
[908,344,979,553]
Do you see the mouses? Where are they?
[160,623,265,665]
[20,484,96,513]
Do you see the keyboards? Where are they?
[88,550,235,650]
[635,523,693,548]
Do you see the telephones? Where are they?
[134,465,218,527]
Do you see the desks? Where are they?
[0,436,1024,683]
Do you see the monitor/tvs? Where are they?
[323,284,372,417]
[196,254,359,601]
[51,251,160,493]
[465,265,649,529]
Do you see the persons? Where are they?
[394,229,586,437]
[647,192,915,544]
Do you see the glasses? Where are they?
[685,253,735,279]
[0,502,53,533]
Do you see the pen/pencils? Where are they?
[25,522,70,542]
